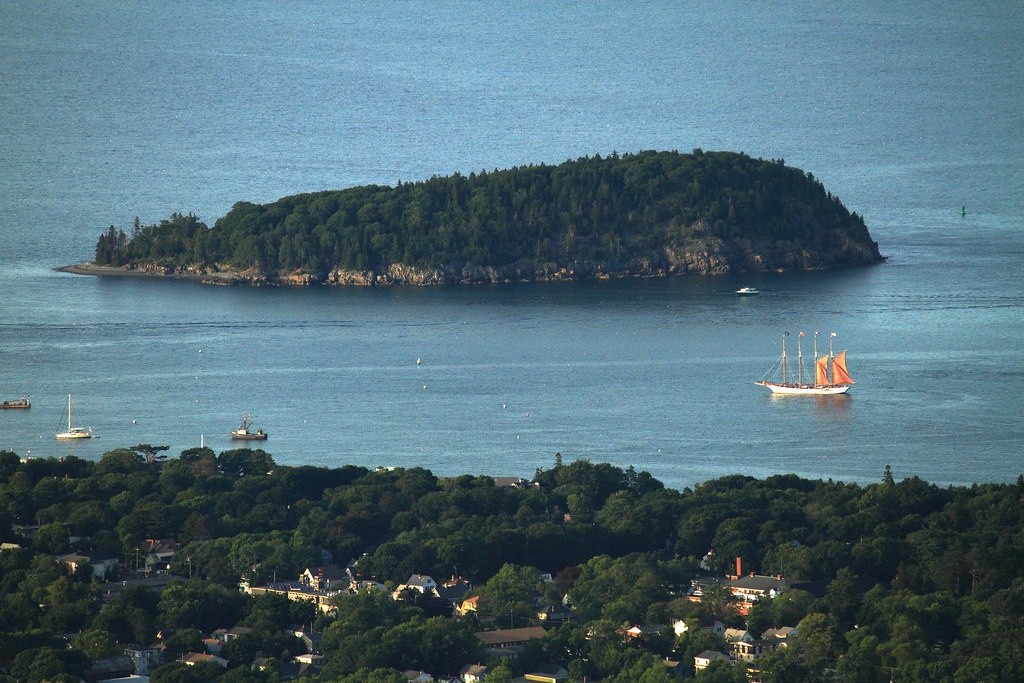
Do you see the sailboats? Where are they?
[53,392,93,441]
[754,329,857,396]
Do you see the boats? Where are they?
[736,287,759,294]
[229,412,269,440]
[0,394,33,410]
